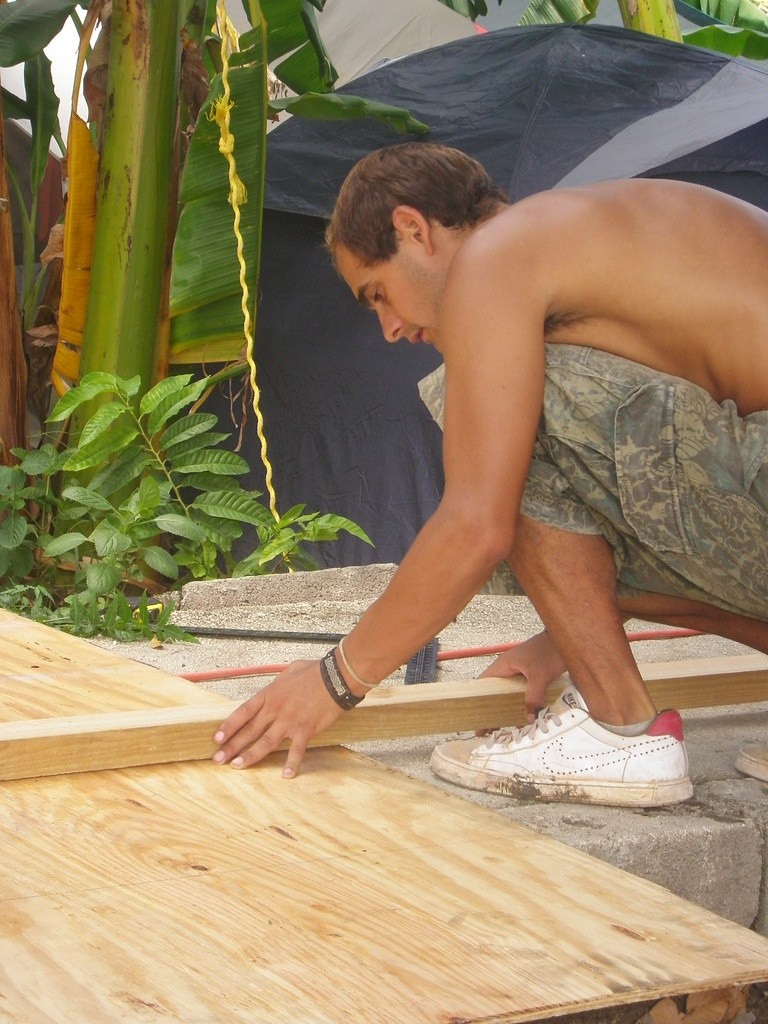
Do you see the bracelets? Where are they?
[319,637,380,713]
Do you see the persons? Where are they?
[213,144,768,806]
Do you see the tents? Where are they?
[166,22,768,595]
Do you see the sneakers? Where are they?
[429,684,693,808]
[734,744,768,783]
